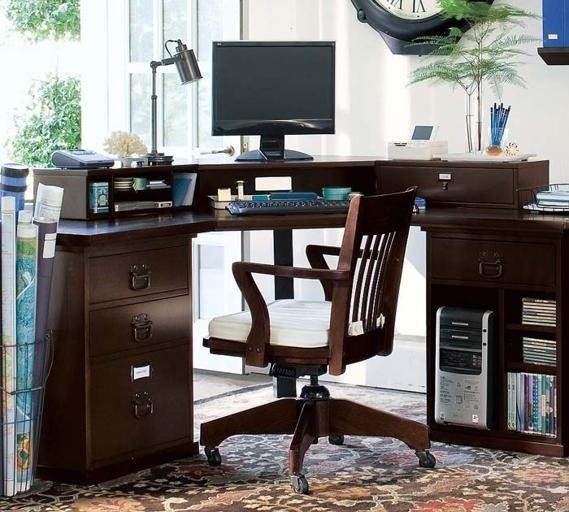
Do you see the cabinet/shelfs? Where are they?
[86,234,192,475]
[426,227,568,458]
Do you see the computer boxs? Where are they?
[435,303,493,430]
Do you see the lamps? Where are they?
[142,38,203,165]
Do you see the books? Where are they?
[523,189,569,212]
[507,297,558,438]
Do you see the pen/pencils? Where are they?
[491,102,511,146]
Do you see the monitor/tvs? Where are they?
[212,41,335,160]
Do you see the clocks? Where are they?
[351,0,494,55]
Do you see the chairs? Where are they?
[200,186,435,493]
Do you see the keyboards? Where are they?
[225,199,358,214]
